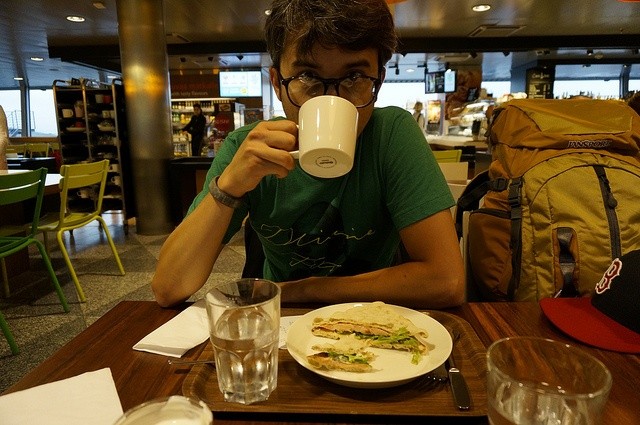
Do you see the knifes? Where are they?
[447,351,473,412]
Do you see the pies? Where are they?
[306,302,436,374]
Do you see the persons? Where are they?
[150,0,465,309]
[412,101,424,130]
[179,102,207,155]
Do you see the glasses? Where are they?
[271,66,382,109]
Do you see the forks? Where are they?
[424,328,460,384]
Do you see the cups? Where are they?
[62,108,74,119]
[76,187,89,198]
[205,279,282,405]
[486,336,612,425]
[289,95,359,178]
[110,175,120,186]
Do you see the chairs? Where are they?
[430,150,461,162]
[26,159,125,303]
[29,143,48,158]
[6,144,27,158]
[434,162,468,183]
[446,183,467,223]
[0,167,72,354]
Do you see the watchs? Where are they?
[208,174,254,214]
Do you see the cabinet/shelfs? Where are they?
[172,98,236,161]
[53,77,125,235]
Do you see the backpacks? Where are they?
[454,98,635,301]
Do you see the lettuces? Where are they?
[317,323,429,372]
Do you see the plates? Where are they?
[66,126,86,132]
[181,307,488,417]
[285,302,453,390]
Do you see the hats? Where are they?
[540,249,640,353]
[413,102,421,110]
